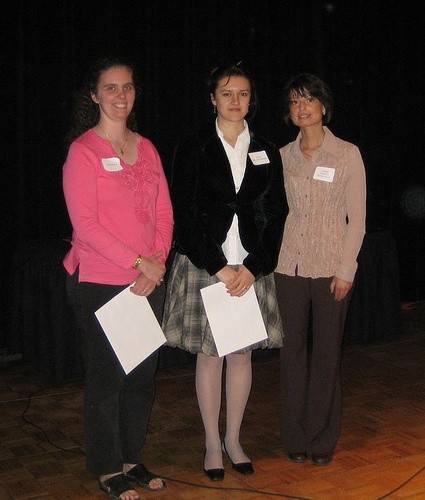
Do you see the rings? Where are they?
[248,286,250,288]
[161,279,163,281]
[144,290,146,291]
[244,286,246,289]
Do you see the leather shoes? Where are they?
[313,455,332,465]
[288,453,305,462]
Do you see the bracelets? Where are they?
[133,255,144,269]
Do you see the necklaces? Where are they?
[97,125,129,155]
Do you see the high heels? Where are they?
[203,446,224,481]
[222,439,252,474]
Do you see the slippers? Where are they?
[125,463,164,491]
[98,473,139,500]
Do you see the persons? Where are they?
[161,61,291,481]
[61,58,175,500]
[277,74,367,463]
